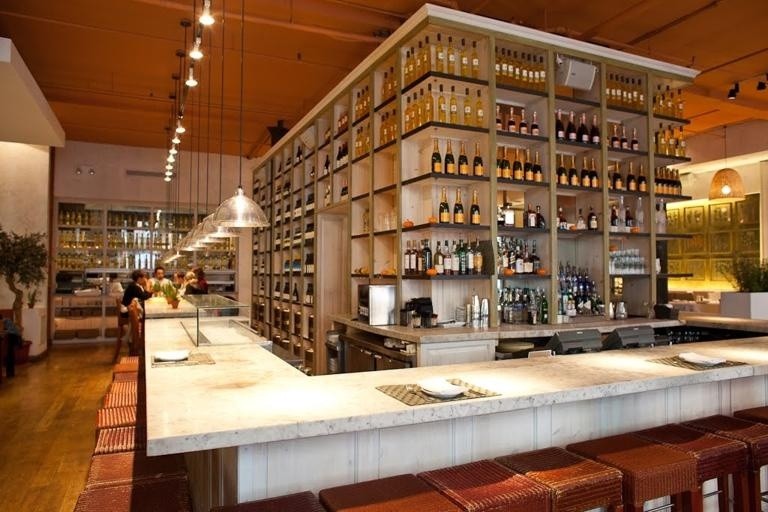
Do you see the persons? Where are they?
[120,270,160,321]
[108,267,207,300]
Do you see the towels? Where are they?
[154,350,189,361]
[417,378,463,395]
[677,352,727,366]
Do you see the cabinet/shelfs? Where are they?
[50,202,237,344]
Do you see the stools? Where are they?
[73,355,188,512]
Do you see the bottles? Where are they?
[197,253,233,271]
[654,122,686,157]
[323,154,330,175]
[434,241,444,274]
[626,161,637,191]
[198,233,235,251]
[403,50,410,85]
[155,231,184,249]
[337,112,348,133]
[274,184,281,202]
[448,85,457,124]
[404,240,410,274]
[431,137,442,172]
[512,147,522,180]
[451,240,459,275]
[59,210,102,226]
[355,123,371,157]
[497,285,547,324]
[398,301,437,328]
[533,151,542,182]
[284,204,289,224]
[282,281,289,301]
[292,199,301,221]
[155,213,191,229]
[530,111,539,136]
[273,282,279,300]
[438,187,449,223]
[305,283,313,305]
[557,205,598,230]
[305,193,313,216]
[377,108,397,145]
[425,83,433,122]
[153,251,191,268]
[436,33,443,73]
[457,141,468,176]
[611,196,645,232]
[497,202,545,228]
[580,156,590,187]
[282,229,289,249]
[106,252,150,271]
[588,158,599,189]
[335,142,348,167]
[106,230,149,249]
[377,206,396,231]
[474,89,484,127]
[106,211,149,227]
[531,55,539,91]
[655,198,666,235]
[416,241,422,274]
[473,238,483,275]
[437,84,445,122]
[457,240,467,274]
[323,185,331,206]
[606,72,645,110]
[519,52,528,88]
[340,180,348,202]
[465,287,489,327]
[496,144,501,178]
[630,127,638,151]
[637,164,647,191]
[555,152,567,184]
[556,108,565,141]
[291,283,298,301]
[409,47,415,84]
[415,41,422,78]
[284,176,289,195]
[501,145,510,179]
[557,262,601,315]
[422,239,431,274]
[608,300,629,320]
[494,45,501,85]
[577,112,590,144]
[453,188,463,224]
[538,56,545,92]
[404,96,411,132]
[495,105,502,130]
[470,40,479,78]
[444,139,455,174]
[418,88,425,127]
[620,126,629,149]
[285,155,291,169]
[460,38,469,76]
[512,51,520,87]
[380,66,395,102]
[507,106,516,131]
[304,223,313,246]
[423,36,430,73]
[524,149,533,181]
[295,145,302,163]
[518,108,527,134]
[56,252,104,270]
[506,50,513,86]
[411,92,418,130]
[284,260,289,276]
[353,85,369,119]
[464,239,474,275]
[497,236,540,274]
[652,84,684,118]
[469,189,479,224]
[500,47,507,85]
[293,227,300,248]
[446,36,455,74]
[463,87,473,126]
[526,54,534,90]
[275,207,280,227]
[607,171,611,189]
[304,252,313,277]
[410,240,418,274]
[308,163,314,181]
[611,160,622,190]
[472,142,483,176]
[609,249,647,274]
[568,155,578,186]
[58,229,102,249]
[611,125,619,150]
[292,259,301,276]
[590,114,600,145]
[566,110,577,143]
[442,241,451,275]
[275,232,280,250]
[323,127,330,142]
[653,165,680,195]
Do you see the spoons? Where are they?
[405,385,431,403]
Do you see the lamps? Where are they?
[161,0,270,263]
[73,164,97,177]
[708,124,746,204]
[728,72,768,101]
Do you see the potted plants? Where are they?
[0,224,56,363]
[717,254,767,320]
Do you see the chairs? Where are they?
[127,297,145,357]
[111,295,129,363]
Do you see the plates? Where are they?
[152,352,189,360]
[679,354,725,367]
[420,387,466,399]
